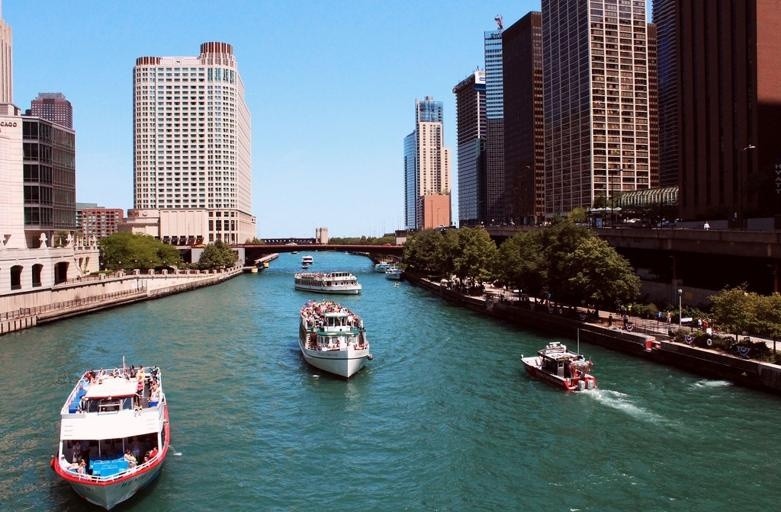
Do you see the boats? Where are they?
[51,356,170,509]
[520,341,597,392]
[301,256,313,268]
[257,263,264,269]
[386,266,402,281]
[298,298,374,379]
[294,271,363,295]
[375,261,391,273]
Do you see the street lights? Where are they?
[678,289,682,326]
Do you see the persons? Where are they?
[701,317,709,331]
[664,309,671,324]
[62,365,160,477]
[608,312,612,328]
[296,271,351,287]
[300,297,368,353]
[623,314,630,326]
[442,275,507,302]
[697,316,702,329]
